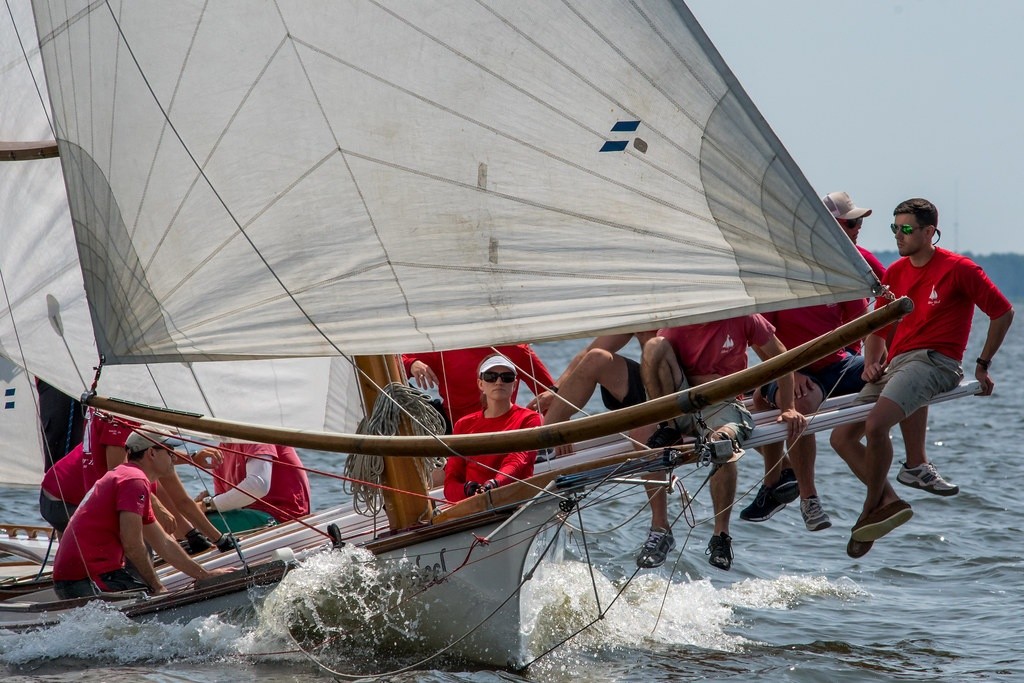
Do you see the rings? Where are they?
[419,374,424,378]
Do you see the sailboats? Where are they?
[0,0,986,671]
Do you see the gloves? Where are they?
[463,478,498,497]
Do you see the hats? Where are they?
[125,425,184,454]
[823,192,872,220]
[479,356,516,375]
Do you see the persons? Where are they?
[740,344,802,523]
[758,189,959,531]
[828,199,1015,560]
[441,354,545,504]
[637,310,810,571]
[524,329,658,463]
[395,341,558,488]
[188,443,312,533]
[34,375,241,606]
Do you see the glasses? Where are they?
[154,446,174,457]
[480,371,515,383]
[846,216,863,228]
[891,223,922,235]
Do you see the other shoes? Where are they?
[535,447,556,462]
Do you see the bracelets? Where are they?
[550,386,559,393]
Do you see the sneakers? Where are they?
[704,532,734,571]
[847,538,874,559]
[636,528,676,569]
[800,496,831,532]
[896,460,959,496]
[850,500,913,542]
[780,469,799,503]
[740,484,786,522]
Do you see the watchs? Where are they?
[977,357,992,369]
[202,496,213,512]
